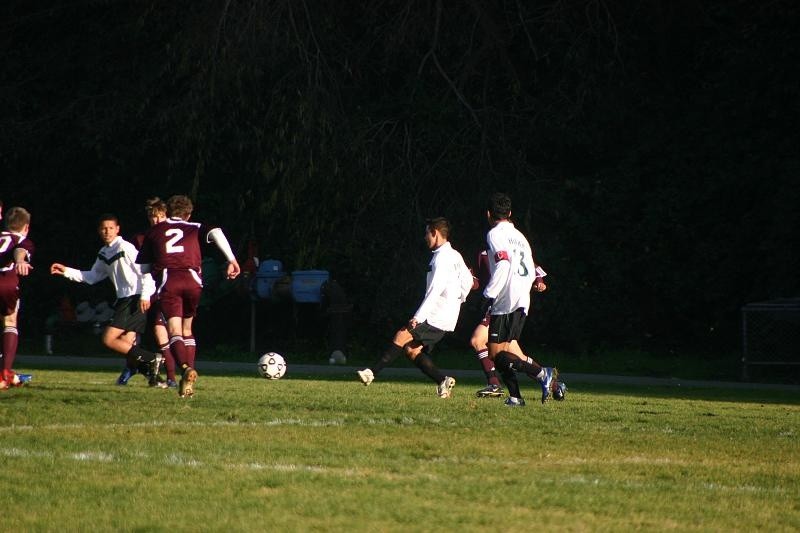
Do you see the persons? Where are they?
[135,196,241,399]
[471,219,568,401]
[0,196,36,391]
[50,214,168,389]
[483,192,559,407]
[134,197,171,389]
[358,216,479,398]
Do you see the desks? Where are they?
[226,276,338,352]
[741,298,800,378]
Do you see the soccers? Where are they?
[258,352,286,379]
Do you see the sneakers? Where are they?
[436,376,455,397]
[166,380,177,388]
[357,368,374,386]
[537,368,552,405]
[504,397,525,406]
[553,382,566,401]
[6,372,32,386]
[117,367,138,385]
[476,386,505,397]
[147,352,162,386]
[179,366,198,398]
[143,370,167,388]
